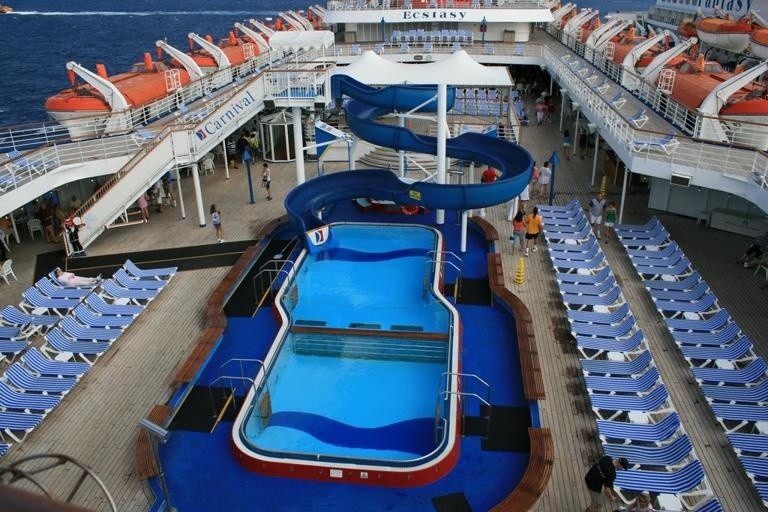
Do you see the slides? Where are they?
[285,74,533,255]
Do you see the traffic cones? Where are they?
[512,257,526,286]
[597,176,607,197]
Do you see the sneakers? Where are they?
[524,251,528,256]
[265,196,269,199]
[598,234,601,239]
[268,197,273,200]
[216,237,226,243]
[532,247,537,252]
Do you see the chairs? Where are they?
[0,149,59,195]
[27,218,46,241]
[454,88,523,118]
[335,1,493,10]
[332,28,527,57]
[199,153,216,176]
[130,67,265,147]
[561,51,680,156]
[0,259,180,458]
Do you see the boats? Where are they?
[44,10,328,144]
[542,0,768,154]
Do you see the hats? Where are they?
[619,457,628,471]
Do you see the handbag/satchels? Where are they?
[262,175,267,181]
[510,234,514,240]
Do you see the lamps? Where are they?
[537,61,601,144]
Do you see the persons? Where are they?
[226,120,261,169]
[53,268,102,287]
[523,207,543,256]
[588,192,607,240]
[602,200,618,244]
[585,130,596,159]
[479,160,552,221]
[577,128,586,160]
[209,203,225,244]
[516,87,554,126]
[734,233,767,264]
[0,193,86,269]
[561,129,571,161]
[261,162,273,202]
[613,492,652,511]
[136,170,178,223]
[511,210,525,255]
[584,454,628,512]
[743,248,767,268]
